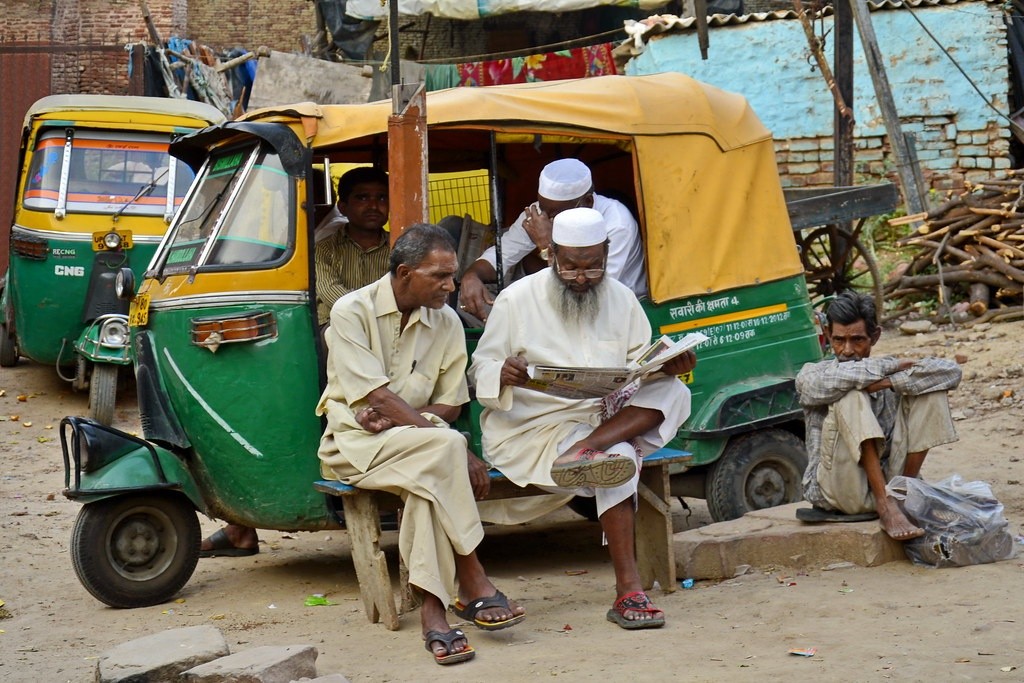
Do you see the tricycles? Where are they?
[59,66,828,609]
[1,94,229,430]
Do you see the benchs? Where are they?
[313,448,695,631]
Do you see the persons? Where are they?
[459,157,648,323]
[315,167,391,361]
[438,216,465,251]
[315,223,527,665]
[794,293,962,540]
[467,206,708,629]
[199,524,260,557]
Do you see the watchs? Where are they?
[540,248,548,261]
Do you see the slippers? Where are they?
[606,591,666,628]
[890,476,1013,567]
[551,447,636,490]
[198,528,259,558]
[454,590,525,631]
[423,628,475,664]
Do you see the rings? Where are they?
[526,216,531,220]
[459,305,465,308]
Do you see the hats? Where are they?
[538,159,592,201]
[551,207,608,247]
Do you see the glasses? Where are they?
[554,255,605,280]
